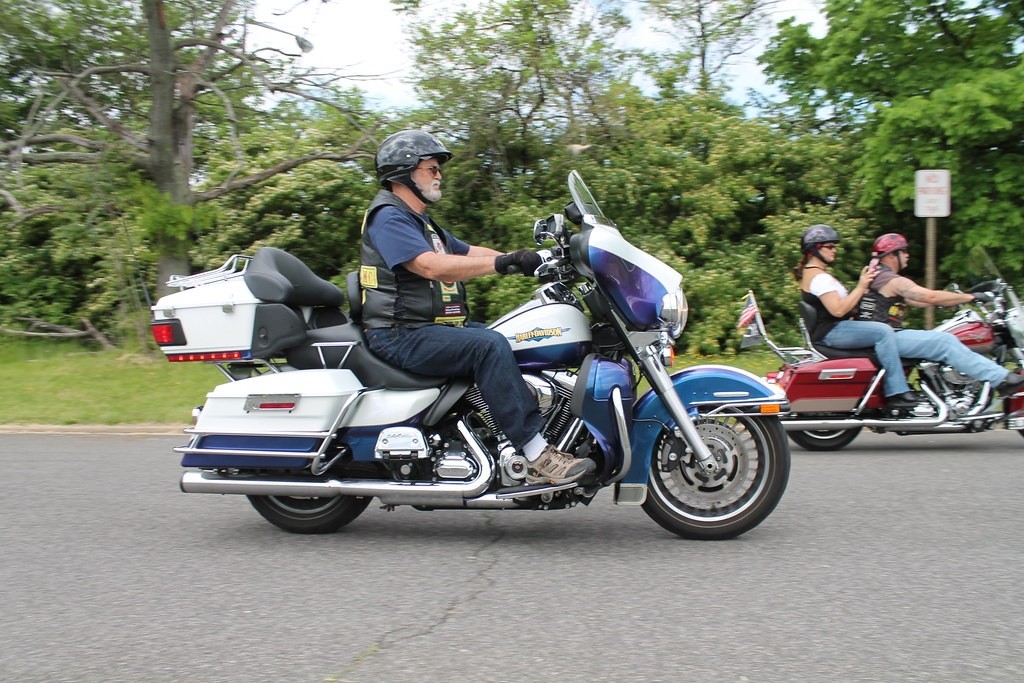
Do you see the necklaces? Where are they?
[804,266,823,269]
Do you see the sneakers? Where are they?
[524,443,596,485]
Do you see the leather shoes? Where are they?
[887,391,931,406]
[997,373,1024,397]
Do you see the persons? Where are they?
[855,232,1024,395]
[359,129,597,486]
[796,224,925,407]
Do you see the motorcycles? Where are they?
[761,246,1024,453]
[150,171,794,541]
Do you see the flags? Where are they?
[737,296,756,329]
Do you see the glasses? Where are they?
[898,249,909,253]
[821,246,833,250]
[415,166,442,176]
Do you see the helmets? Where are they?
[801,225,840,247]
[374,129,454,192]
[873,233,908,252]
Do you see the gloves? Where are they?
[495,249,546,277]
